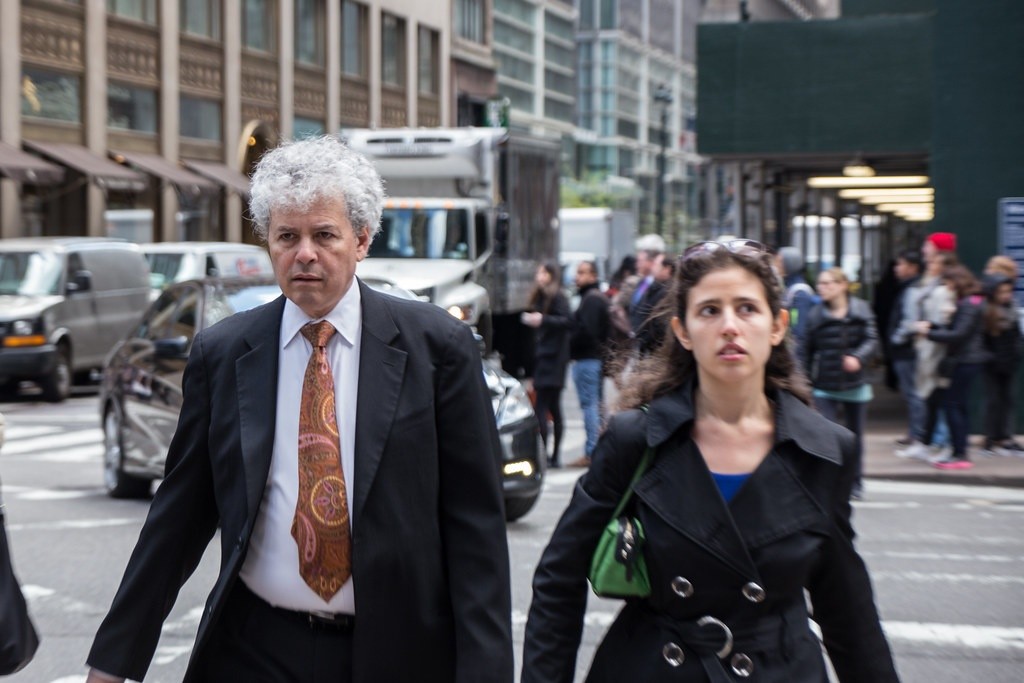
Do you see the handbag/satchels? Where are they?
[587,402,655,602]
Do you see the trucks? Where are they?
[323,126,560,359]
[561,206,637,316]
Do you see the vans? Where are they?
[0,237,275,404]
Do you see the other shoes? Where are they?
[934,454,973,470]
[894,439,933,463]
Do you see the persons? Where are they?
[779,248,877,498]
[84,137,515,683]
[520,248,900,683]
[523,235,678,468]
[892,232,1024,467]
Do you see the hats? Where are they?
[926,232,959,254]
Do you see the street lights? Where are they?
[653,83,674,238]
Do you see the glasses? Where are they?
[679,238,769,258]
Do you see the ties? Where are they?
[290,320,353,603]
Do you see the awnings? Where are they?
[184,159,251,196]
[0,142,63,182]
[23,138,147,190]
[107,149,219,197]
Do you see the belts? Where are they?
[242,587,357,634]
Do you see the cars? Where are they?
[100,276,549,523]
